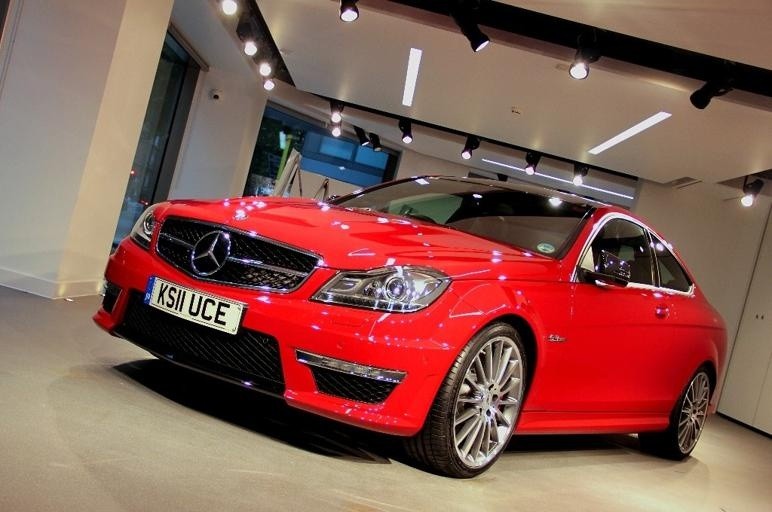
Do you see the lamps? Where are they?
[460,135,589,187]
[740,175,764,208]
[338,0,736,111]
[328,100,415,154]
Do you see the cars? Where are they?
[91,173,729,479]
